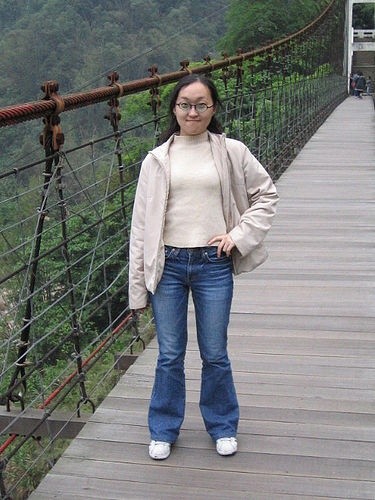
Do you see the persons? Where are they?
[351,70,373,99]
[128,75,280,461]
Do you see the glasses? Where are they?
[175,101,215,113]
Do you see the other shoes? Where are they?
[215,436,238,456]
[148,440,172,459]
